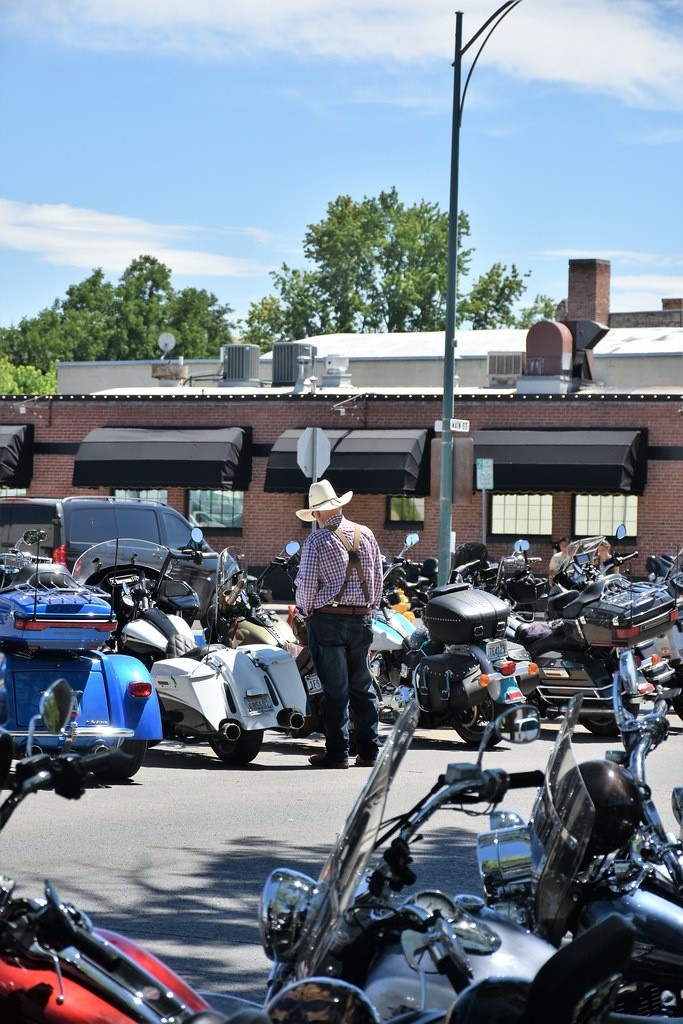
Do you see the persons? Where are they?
[550,538,620,588]
[295,479,382,770]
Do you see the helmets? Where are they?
[556,760,643,856]
[505,576,547,605]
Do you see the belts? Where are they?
[316,606,371,614]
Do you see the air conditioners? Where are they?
[486,351,526,383]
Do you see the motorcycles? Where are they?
[0,522,683,1024]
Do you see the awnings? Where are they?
[0,423,32,489]
[71,427,253,492]
[263,426,436,497]
[473,427,649,497]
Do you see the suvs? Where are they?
[0,494,248,642]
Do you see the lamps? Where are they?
[330,394,362,416]
[11,394,48,414]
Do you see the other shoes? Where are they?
[355,756,375,767]
[309,754,349,769]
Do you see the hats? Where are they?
[295,479,353,522]
